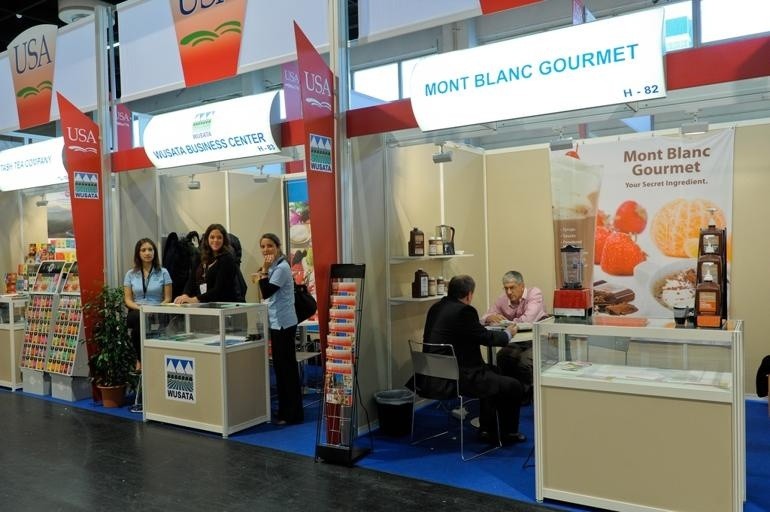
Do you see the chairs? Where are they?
[409,339,501,461]
[295,351,321,406]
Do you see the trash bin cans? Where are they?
[373,390,416,444]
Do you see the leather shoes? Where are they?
[274,420,288,425]
[510,433,527,442]
[272,411,279,415]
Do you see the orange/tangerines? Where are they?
[650,198,727,258]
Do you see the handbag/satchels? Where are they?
[278,258,317,324]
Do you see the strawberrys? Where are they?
[594,226,610,264]
[596,209,607,226]
[600,231,648,276]
[613,200,647,234]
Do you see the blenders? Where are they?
[437,225,455,254]
[553,244,592,323]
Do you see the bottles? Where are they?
[408,227,424,257]
[412,268,429,299]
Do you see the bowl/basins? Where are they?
[648,258,700,317]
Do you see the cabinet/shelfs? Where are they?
[531,317,747,511]
[315,263,369,465]
[139,302,271,438]
[389,250,475,305]
[0,295,31,391]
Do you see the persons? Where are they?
[250,233,305,426]
[479,271,550,406]
[173,223,235,305]
[419,274,524,447]
[123,237,173,374]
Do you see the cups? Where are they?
[674,306,688,328]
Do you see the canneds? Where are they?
[436,276,445,295]
[435,237,444,255]
[428,237,436,256]
[428,277,437,296]
